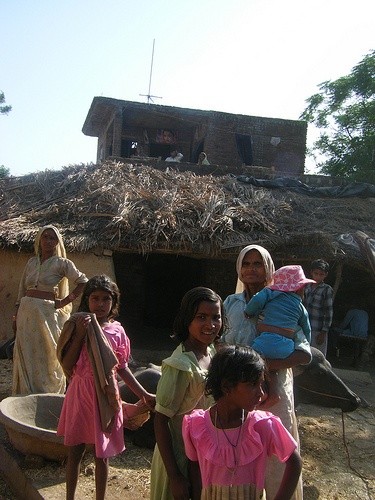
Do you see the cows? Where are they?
[119,345,361,452]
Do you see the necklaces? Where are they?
[215,408,245,488]
[217,409,242,467]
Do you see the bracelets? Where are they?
[60,300,64,307]
[68,293,77,302]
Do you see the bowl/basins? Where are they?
[0,393,92,460]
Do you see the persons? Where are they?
[12,224,89,394]
[302,259,334,359]
[198,152,210,164]
[182,344,303,500]
[165,148,184,163]
[327,297,368,359]
[150,287,230,500]
[223,244,312,500]
[56,274,156,500]
[244,265,311,410]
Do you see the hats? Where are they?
[266,264,317,293]
